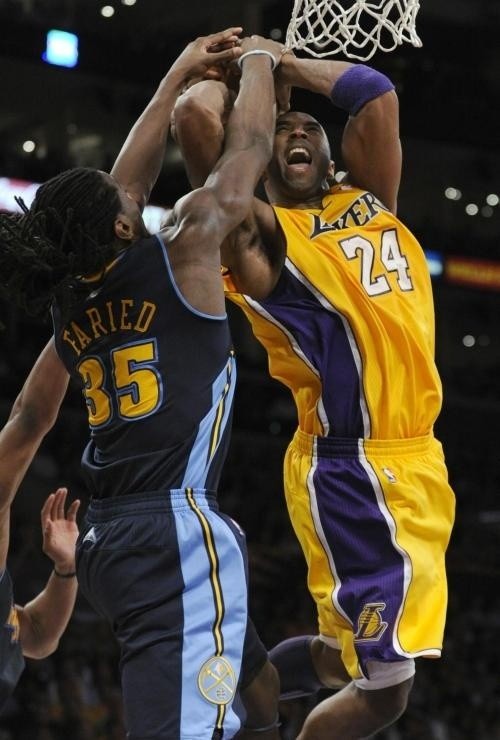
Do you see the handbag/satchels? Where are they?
[54,568,77,578]
[238,50,277,72]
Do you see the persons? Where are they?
[172,32,460,740]
[0,336,82,702]
[20,26,279,740]
